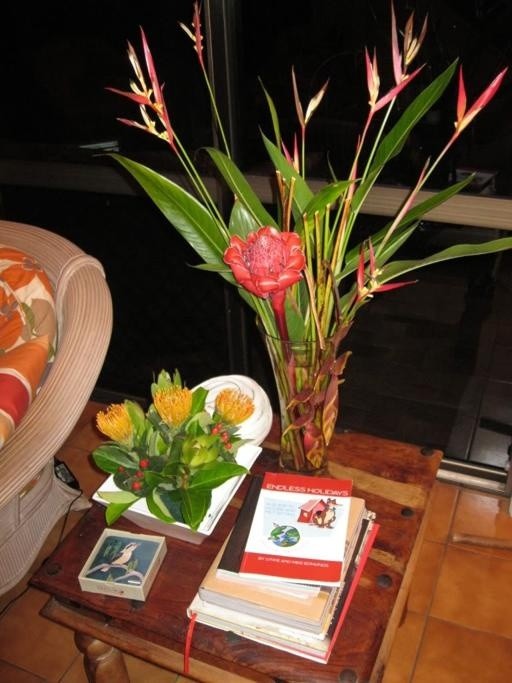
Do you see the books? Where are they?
[184,472,382,665]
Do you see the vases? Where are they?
[255,315,336,473]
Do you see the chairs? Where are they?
[0,217,115,598]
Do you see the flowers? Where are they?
[105,0,512,337]
[87,369,256,534]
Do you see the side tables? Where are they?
[28,414,445,683]
